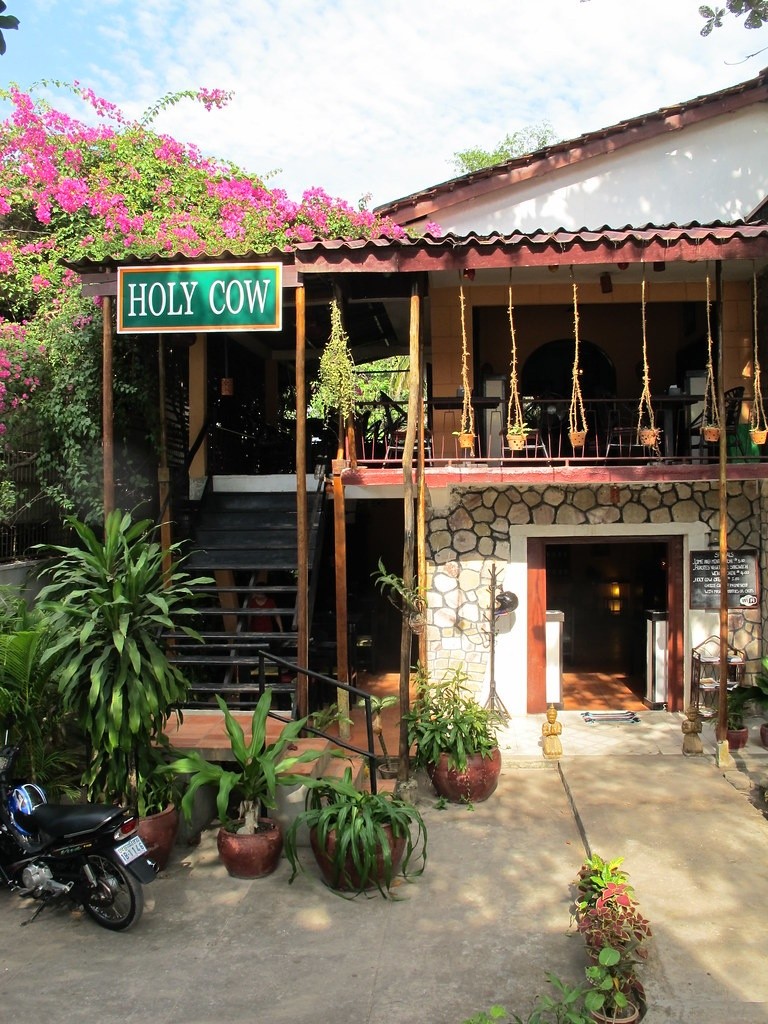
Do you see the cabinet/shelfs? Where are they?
[692,635,745,719]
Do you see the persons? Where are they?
[247,580,284,631]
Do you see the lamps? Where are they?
[600,272,612,293]
[222,342,233,396]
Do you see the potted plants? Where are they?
[748,428,768,445]
[506,423,532,450]
[638,425,664,445]
[23,500,217,872]
[700,423,721,442]
[704,657,768,750]
[401,659,508,811]
[149,686,355,879]
[356,695,400,779]
[282,767,428,902]
[452,421,476,448]
[568,430,586,446]
[460,854,653,1024]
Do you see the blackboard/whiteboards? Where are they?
[689,550,759,609]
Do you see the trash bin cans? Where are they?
[355,635,378,675]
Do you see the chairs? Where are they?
[605,427,654,465]
[379,391,434,467]
[498,426,553,467]
[678,386,748,465]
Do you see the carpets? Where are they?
[581,711,641,723]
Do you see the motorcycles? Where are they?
[1,730,162,933]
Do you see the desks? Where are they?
[251,597,380,711]
